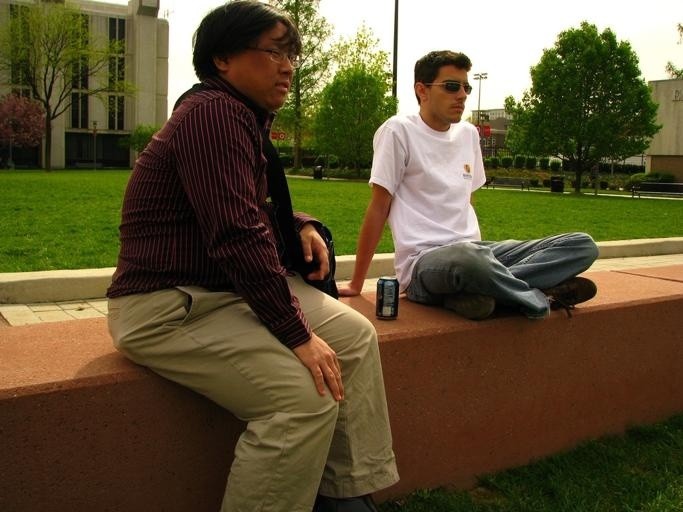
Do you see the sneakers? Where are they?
[316,495,376,512]
[543,275,596,319]
[441,293,498,321]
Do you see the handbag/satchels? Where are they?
[281,226,338,300]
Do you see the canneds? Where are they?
[376,276,399,320]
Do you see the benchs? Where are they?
[629,182,682,200]
[487,175,530,191]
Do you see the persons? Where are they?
[337,51,598,320]
[106,0,400,512]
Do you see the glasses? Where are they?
[424,80,471,93]
[245,42,305,71]
